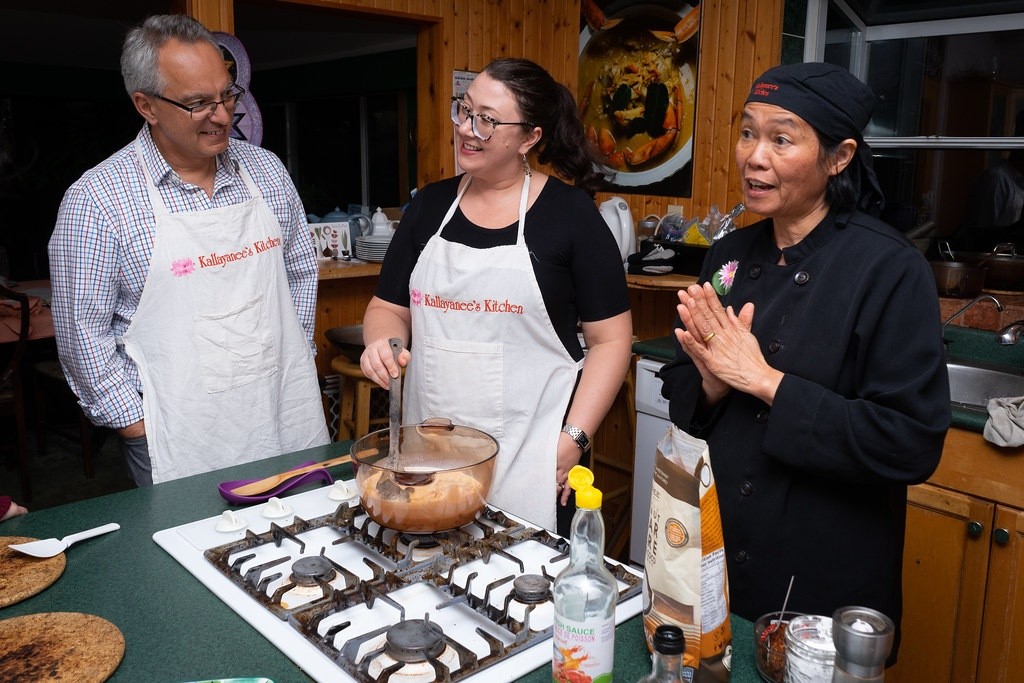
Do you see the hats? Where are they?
[745,62,881,143]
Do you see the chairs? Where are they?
[0,285,94,504]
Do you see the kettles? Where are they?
[599,195,637,273]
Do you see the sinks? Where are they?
[945,358,1024,413]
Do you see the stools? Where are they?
[332,354,406,455]
[583,334,639,502]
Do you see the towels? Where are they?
[983,395,1024,448]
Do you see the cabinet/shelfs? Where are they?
[885,427,1024,683]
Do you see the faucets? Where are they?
[994,319,1024,346]
[942,294,1003,354]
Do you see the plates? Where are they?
[355,237,392,263]
[578,26,694,187]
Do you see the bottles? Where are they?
[636,624,690,683]
[638,220,658,239]
[551,465,619,683]
[832,606,894,683]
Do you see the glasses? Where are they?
[143,84,244,123]
[451,96,535,141]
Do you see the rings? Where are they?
[556,483,563,489]
[704,332,716,342]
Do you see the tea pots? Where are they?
[358,206,400,236]
[306,206,361,246]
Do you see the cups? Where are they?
[753,610,806,683]
[783,615,837,683]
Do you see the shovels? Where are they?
[229,448,378,496]
[376,337,411,500]
[7,520,120,559]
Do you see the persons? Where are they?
[0,497,28,522]
[659,59,952,670]
[970,125,1024,256]
[47,13,319,486]
[360,54,632,541]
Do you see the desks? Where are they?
[0,280,55,344]
[315,254,699,292]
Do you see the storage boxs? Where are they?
[639,231,706,277]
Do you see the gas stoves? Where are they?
[153,477,646,683]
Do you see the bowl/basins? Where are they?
[929,260,984,299]
[349,416,500,532]
[943,249,1024,292]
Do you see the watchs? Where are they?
[562,423,591,453]
